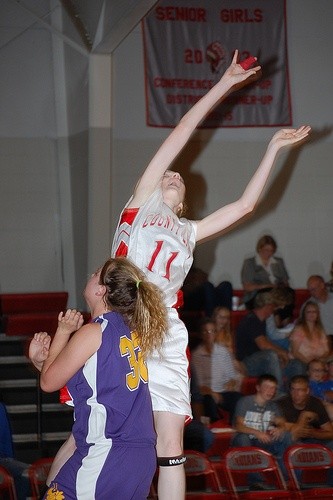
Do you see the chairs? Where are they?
[286,443,333,500]
[30,458,54,500]
[0,469,16,500]
[147,450,228,500]
[223,447,290,500]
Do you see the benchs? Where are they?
[0,289,312,487]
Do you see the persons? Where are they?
[233,287,333,400]
[111,50,310,500]
[181,267,233,318]
[212,306,246,375]
[29,258,172,500]
[276,374,333,489]
[305,359,333,420]
[190,321,243,427]
[183,363,214,454]
[241,234,290,302]
[300,275,333,338]
[230,373,302,491]
